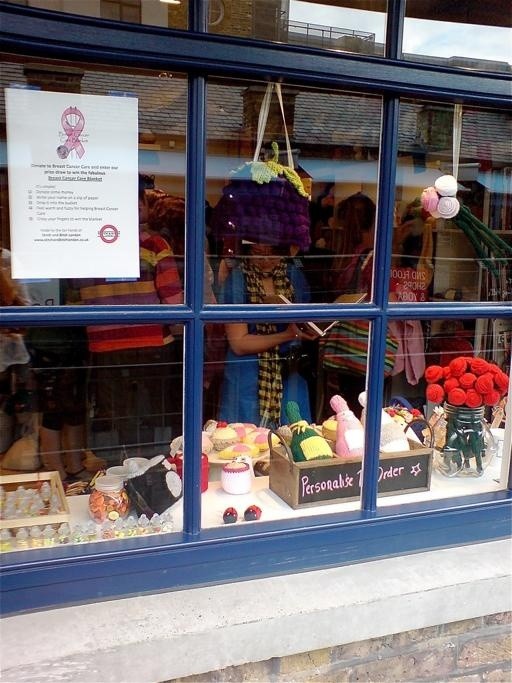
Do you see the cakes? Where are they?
[222,461,253,494]
[202,420,280,459]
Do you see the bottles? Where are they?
[88,458,150,521]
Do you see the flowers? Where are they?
[422,175,512,276]
[426,357,509,471]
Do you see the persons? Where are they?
[2,168,437,484]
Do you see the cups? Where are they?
[167,452,208,490]
[220,459,255,495]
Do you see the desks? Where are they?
[66,427,504,544]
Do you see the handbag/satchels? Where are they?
[321,321,397,378]
[223,159,311,249]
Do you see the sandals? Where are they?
[64,467,91,479]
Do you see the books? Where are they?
[472,165,512,375]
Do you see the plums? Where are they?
[244,505,262,520]
[223,507,237,524]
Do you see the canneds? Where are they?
[89,457,149,525]
